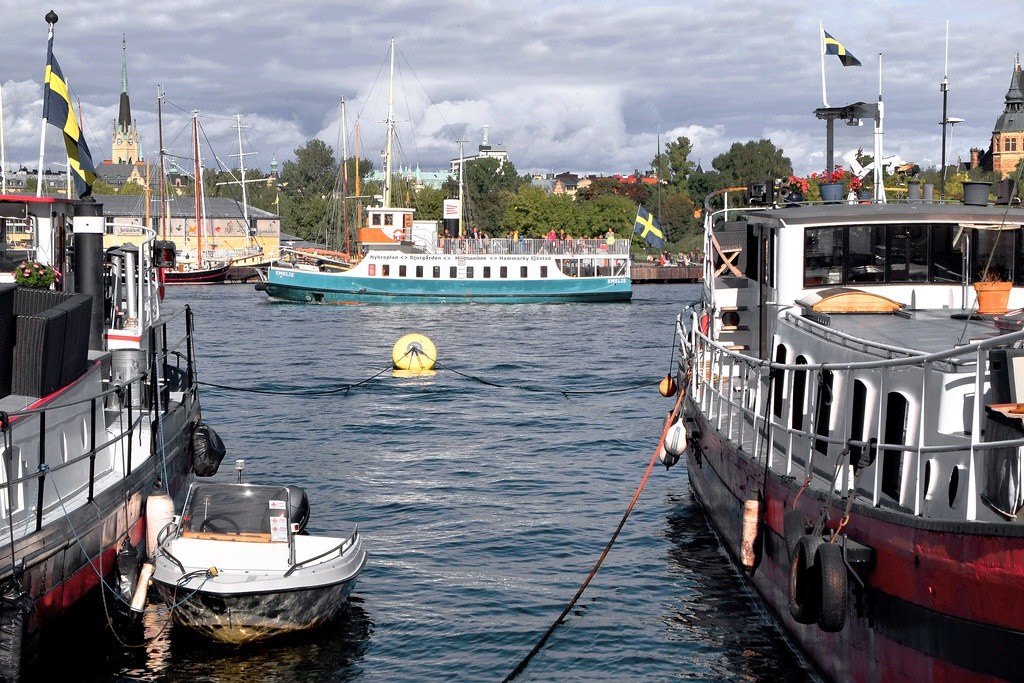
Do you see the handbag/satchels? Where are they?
[607,235,615,245]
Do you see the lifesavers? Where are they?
[393,229,403,240]
[783,508,848,632]
[699,309,708,349]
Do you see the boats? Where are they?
[661,16,1023,682]
[0,34,224,682]
[146,474,371,644]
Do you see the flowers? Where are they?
[779,175,810,198]
[812,164,845,184]
[11,260,62,287]
[846,172,866,193]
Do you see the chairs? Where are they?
[712,233,743,278]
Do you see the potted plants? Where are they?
[973,268,1013,310]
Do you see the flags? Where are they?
[634,205,667,250]
[43,38,98,199]
[823,29,862,67]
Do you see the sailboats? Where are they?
[105,81,280,283]
[254,35,634,305]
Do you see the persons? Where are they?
[677,245,704,266]
[606,228,615,252]
[563,260,568,274]
[536,230,605,255]
[290,257,296,268]
[506,230,526,253]
[648,250,672,266]
[573,264,600,277]
[304,254,308,264]
[439,228,490,254]
[321,264,327,271]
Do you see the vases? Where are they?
[857,192,872,199]
[818,182,845,203]
[960,181,994,206]
[784,193,803,207]
